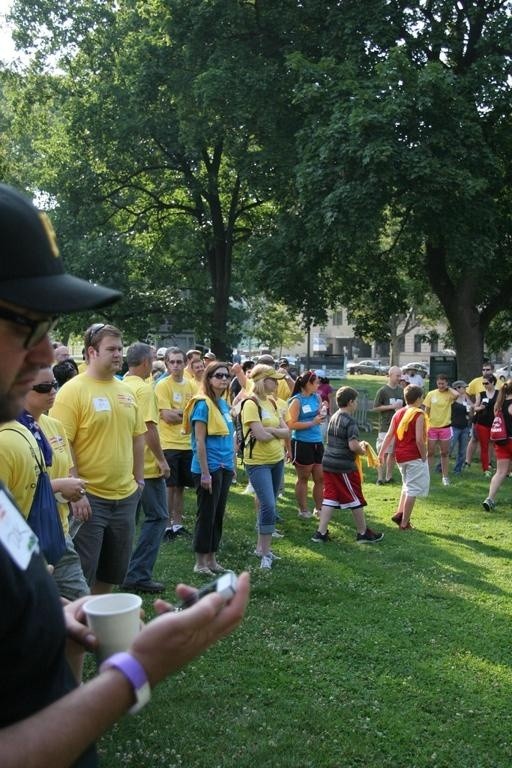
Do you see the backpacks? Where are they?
[0,428,66,566]
[489,407,507,441]
[236,396,278,457]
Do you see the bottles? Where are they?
[318,405,327,424]
[68,512,83,538]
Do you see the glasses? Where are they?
[32,381,60,393]
[482,382,489,385]
[89,323,104,343]
[0,306,60,350]
[211,373,231,379]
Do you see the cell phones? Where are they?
[159,572,237,616]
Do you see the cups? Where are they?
[82,592,146,669]
[395,398,403,412]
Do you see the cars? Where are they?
[240,353,297,368]
[354,360,391,376]
[493,366,512,380]
[401,361,430,379]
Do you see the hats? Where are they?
[253,368,286,382]
[155,347,168,358]
[278,358,288,367]
[203,352,217,361]
[314,369,327,379]
[0,187,124,315]
[451,380,468,389]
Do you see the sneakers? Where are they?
[436,461,512,486]
[385,477,397,484]
[164,506,384,576]
[376,479,384,484]
[392,513,411,528]
[482,497,495,512]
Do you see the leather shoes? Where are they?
[133,578,165,594]
